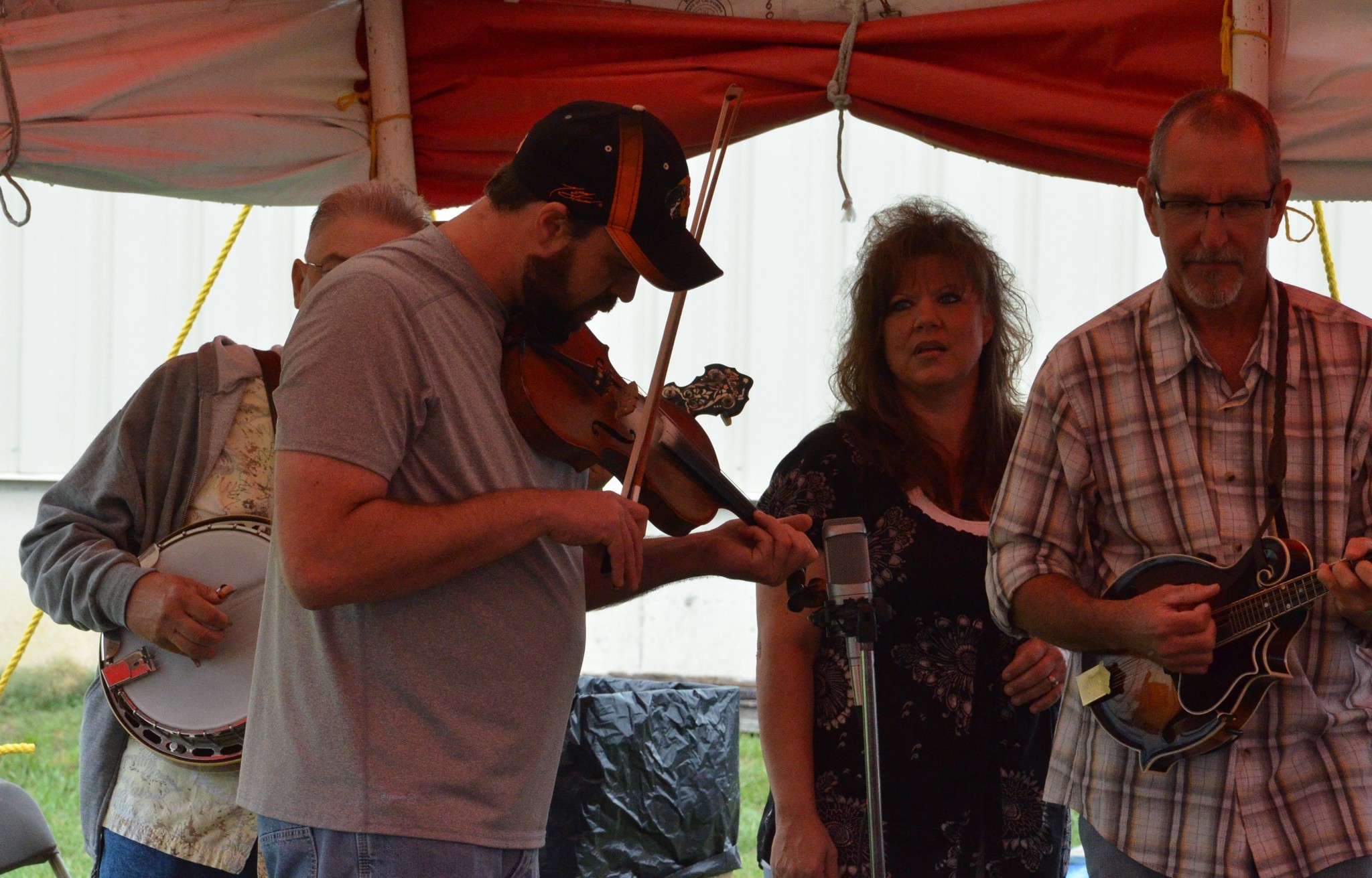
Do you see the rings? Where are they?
[1048,675,1059,688]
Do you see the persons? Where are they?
[756,193,1080,878]
[984,88,1371,878]
[237,99,818,878]
[20,180,434,878]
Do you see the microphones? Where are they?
[820,517,875,609]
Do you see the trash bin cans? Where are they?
[541,675,741,878]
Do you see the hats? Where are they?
[516,100,724,292]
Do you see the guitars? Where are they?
[97,362,754,770]
[1078,534,1372,775]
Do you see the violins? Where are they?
[499,320,832,612]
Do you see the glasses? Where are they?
[1152,179,1277,219]
[306,261,339,277]
